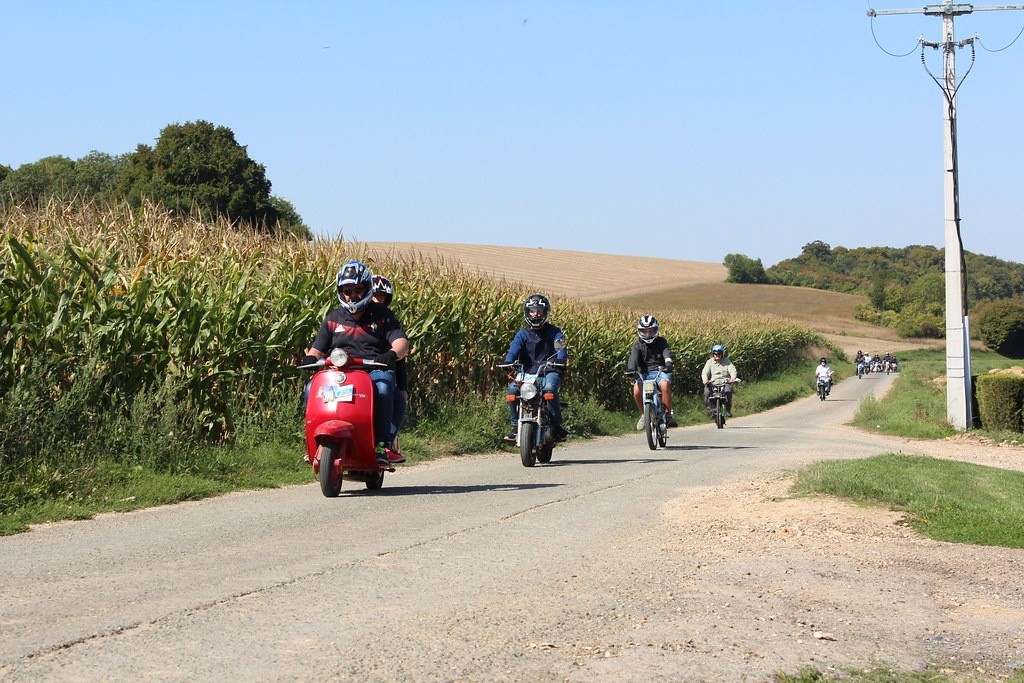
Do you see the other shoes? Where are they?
[636,417,650,431]
[376,456,396,471]
[725,409,732,417]
[666,416,677,427]
[304,455,308,462]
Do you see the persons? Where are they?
[854,350,897,375]
[295,261,408,465]
[815,358,832,395]
[701,345,737,418]
[495,294,572,441]
[625,316,678,431]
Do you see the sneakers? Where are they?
[383,449,406,464]
[503,428,518,440]
[552,428,570,440]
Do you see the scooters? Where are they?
[816,376,830,401]
[856,359,898,379]
[496,360,567,469]
[288,347,401,497]
[707,379,731,429]
[622,366,673,451]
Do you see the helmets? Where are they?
[636,315,659,345]
[525,295,550,328]
[371,275,393,307]
[336,262,374,313]
[711,345,724,360]
[820,358,826,363]
[857,350,896,358]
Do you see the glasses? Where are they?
[339,287,365,297]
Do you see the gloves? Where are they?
[551,359,566,371]
[501,362,512,372]
[662,367,671,374]
[374,350,398,371]
[302,355,320,371]
[626,368,635,377]
